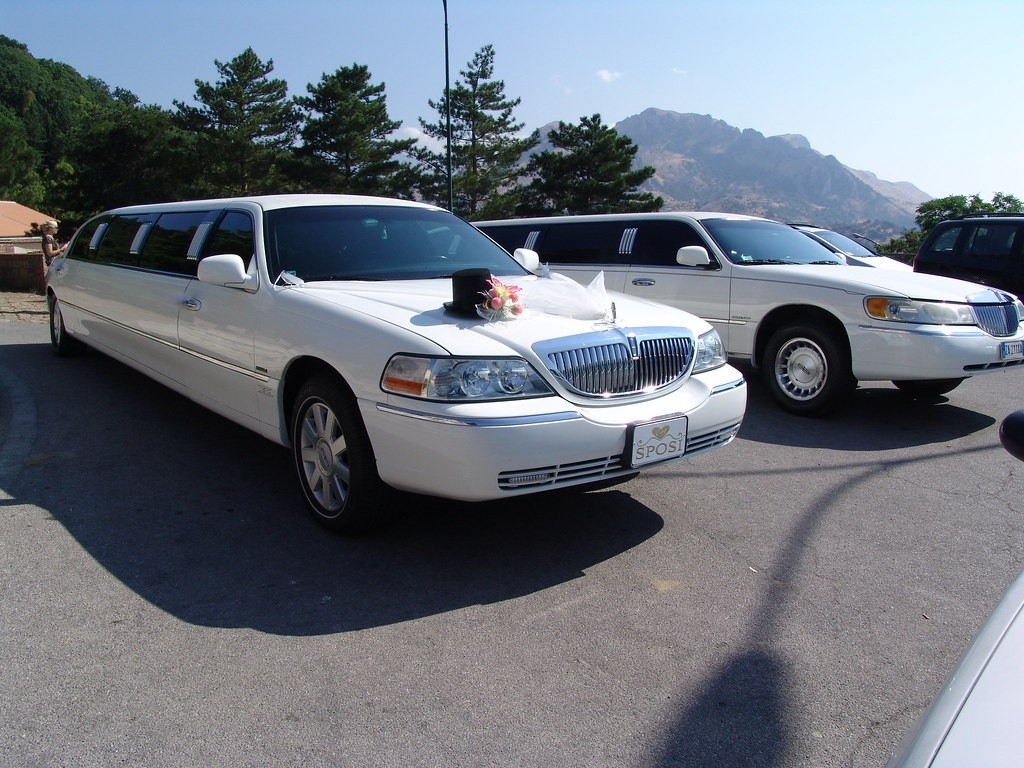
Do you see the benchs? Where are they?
[969,236,1011,256]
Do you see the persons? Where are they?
[42,220,68,268]
[24,222,41,237]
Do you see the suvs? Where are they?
[913,213,1024,306]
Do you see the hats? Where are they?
[46,221,58,228]
[443,268,503,319]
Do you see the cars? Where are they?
[451,210,1024,416]
[788,222,914,272]
[45,192,745,534]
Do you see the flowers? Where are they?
[475,274,523,328]
